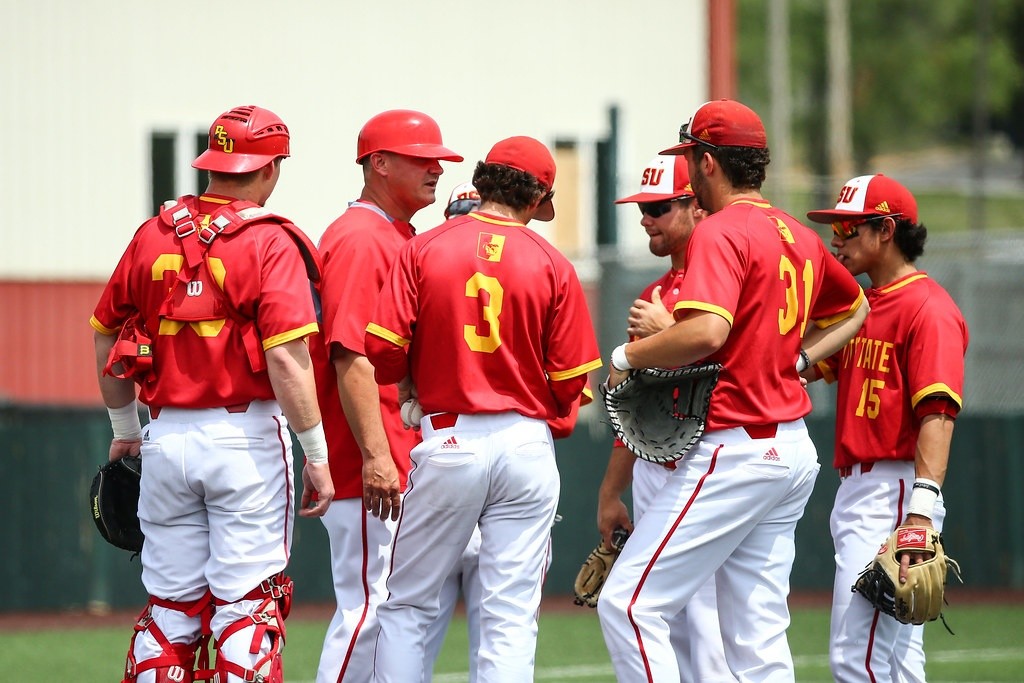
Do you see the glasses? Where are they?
[679,124,718,151]
[831,213,903,240]
[637,194,694,219]
[446,199,484,214]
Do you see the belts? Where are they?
[743,421,780,440]
[431,413,459,430]
[657,460,678,471]
[839,461,875,480]
[148,403,250,420]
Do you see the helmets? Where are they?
[193,106,289,173]
[357,111,463,165]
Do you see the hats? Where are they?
[615,155,695,205]
[444,184,482,219]
[657,98,767,156]
[806,174,919,224]
[485,136,557,222]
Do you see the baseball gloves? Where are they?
[89,454,145,554]
[597,362,724,463]
[572,520,633,608]
[851,524,949,627]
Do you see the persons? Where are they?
[363,135,604,683]
[303,109,483,683]
[798,172,970,683]
[596,100,869,683]
[89,105,336,683]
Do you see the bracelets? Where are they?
[294,420,330,464]
[905,478,941,520]
[610,342,633,372]
[106,398,143,441]
[796,347,811,373]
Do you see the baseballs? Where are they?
[400,397,424,427]
